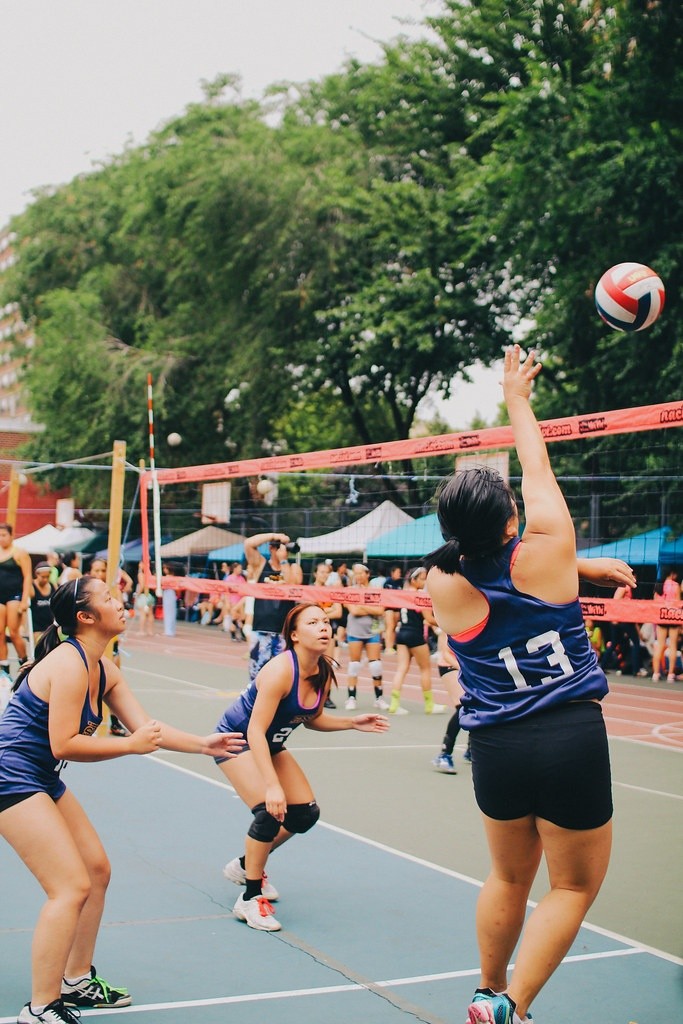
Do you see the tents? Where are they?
[297,500,448,578]
[576,526,683,588]
[11,524,297,622]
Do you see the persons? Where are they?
[212,602,390,933]
[0,574,246,1024]
[426,345,637,1024]
[0,524,32,677]
[28,549,133,737]
[244,533,302,682]
[584,562,683,683]
[133,560,248,643]
[301,562,473,775]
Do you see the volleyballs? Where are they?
[595,257,664,335]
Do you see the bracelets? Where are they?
[279,560,288,565]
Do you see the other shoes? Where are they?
[462,749,473,764]
[636,667,683,681]
[344,696,358,710]
[383,648,397,655]
[388,706,410,715]
[424,703,450,714]
[372,696,389,709]
[324,698,336,708]
[432,753,457,774]
[109,725,132,737]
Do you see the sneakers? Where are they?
[223,855,279,900]
[232,891,282,931]
[465,988,536,1024]
[60,966,133,1008]
[17,998,82,1024]
